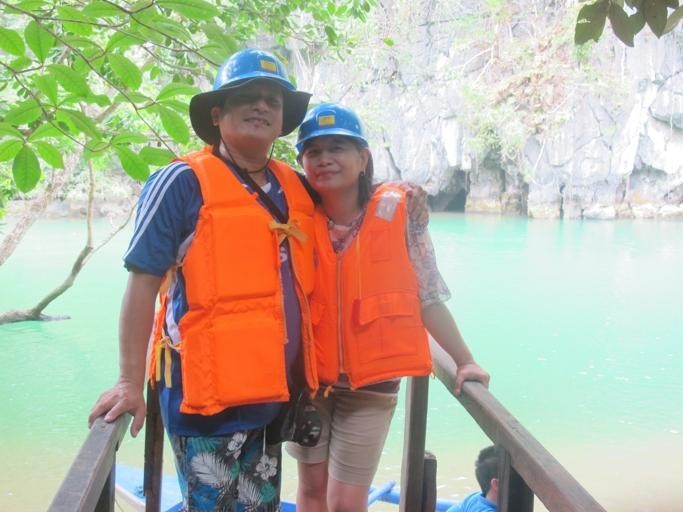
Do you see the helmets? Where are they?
[213,47,296,91]
[295,103,368,152]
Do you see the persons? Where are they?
[443,444,500,512]
[85,48,430,512]
[281,101,490,512]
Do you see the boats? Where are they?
[112,460,298,511]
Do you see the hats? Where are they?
[190,76,313,145]
